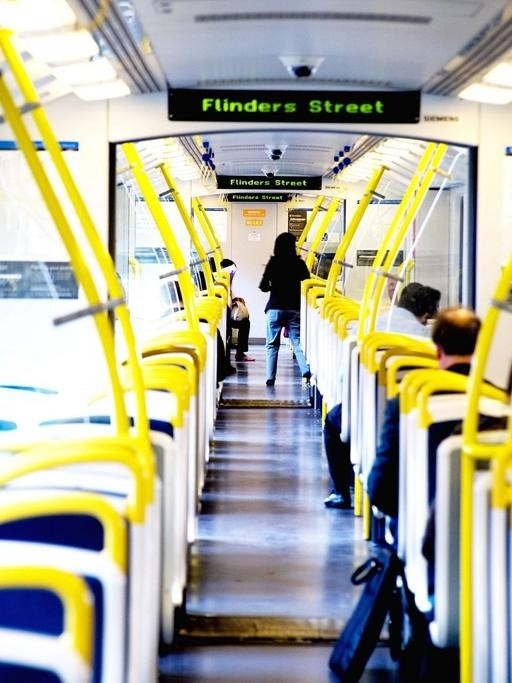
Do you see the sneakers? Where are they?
[235,354,255,361]
[325,494,348,508]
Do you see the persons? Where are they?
[158,258,256,388]
[365,307,506,543]
[258,232,311,386]
[323,282,441,508]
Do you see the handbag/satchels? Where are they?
[330,547,397,683]
[230,301,248,321]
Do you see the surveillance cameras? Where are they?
[265,144,288,160]
[262,167,278,177]
[277,56,326,80]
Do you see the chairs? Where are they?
[0,270,225,681]
[290,280,512,682]
